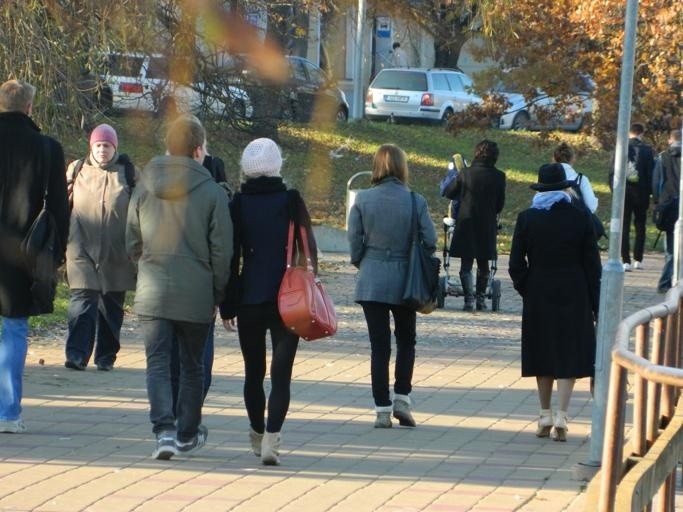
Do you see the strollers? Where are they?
[438,179,501,311]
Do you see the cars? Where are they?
[484,70,602,135]
[84,54,255,122]
[365,65,479,128]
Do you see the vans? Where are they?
[228,55,348,124]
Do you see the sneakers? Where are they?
[145,425,176,462]
[94,353,116,372]
[441,217,454,227]
[176,423,210,458]
[62,348,89,372]
[634,259,643,270]
[623,262,633,273]
[0,416,29,435]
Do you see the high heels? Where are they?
[534,406,554,440]
[552,410,571,443]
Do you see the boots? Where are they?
[246,423,262,457]
[475,268,491,311]
[258,429,284,469]
[457,269,474,312]
[373,404,392,430]
[391,393,417,428]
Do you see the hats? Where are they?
[89,124,118,149]
[237,136,285,180]
[528,162,574,193]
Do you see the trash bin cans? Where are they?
[346,170,374,231]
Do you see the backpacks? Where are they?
[566,172,610,243]
[610,143,642,192]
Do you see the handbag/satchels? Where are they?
[652,177,680,232]
[401,246,445,316]
[275,267,339,343]
[17,199,70,314]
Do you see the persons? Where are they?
[393,42,400,67]
[609,123,654,271]
[165,114,227,424]
[348,144,440,428]
[124,120,233,460]
[442,141,505,310]
[509,164,601,442]
[554,142,599,214]
[66,124,142,371]
[0,79,70,432]
[652,130,683,293]
[221,138,337,466]
